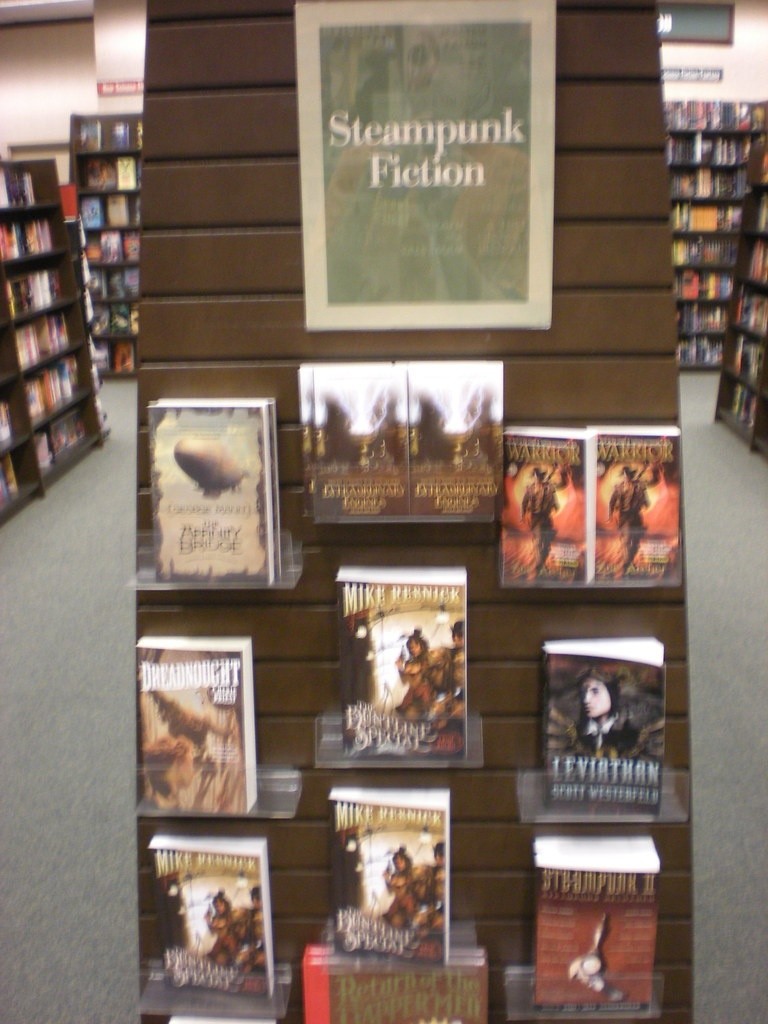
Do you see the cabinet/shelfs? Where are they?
[65,218,111,439]
[665,130,768,371]
[502,529,691,1022]
[713,145,768,451]
[0,159,102,498]
[0,318,46,526]
[126,528,305,1020]
[315,462,497,974]
[68,114,144,381]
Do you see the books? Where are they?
[297,358,506,516]
[534,833,661,1015]
[591,421,682,591]
[145,396,285,583]
[302,784,487,1024]
[336,567,471,767]
[136,637,259,816]
[542,633,665,816]
[663,101,768,429]
[498,426,594,586]
[149,836,279,1024]
[0,117,143,510]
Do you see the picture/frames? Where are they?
[657,2,733,44]
[294,0,557,333]
